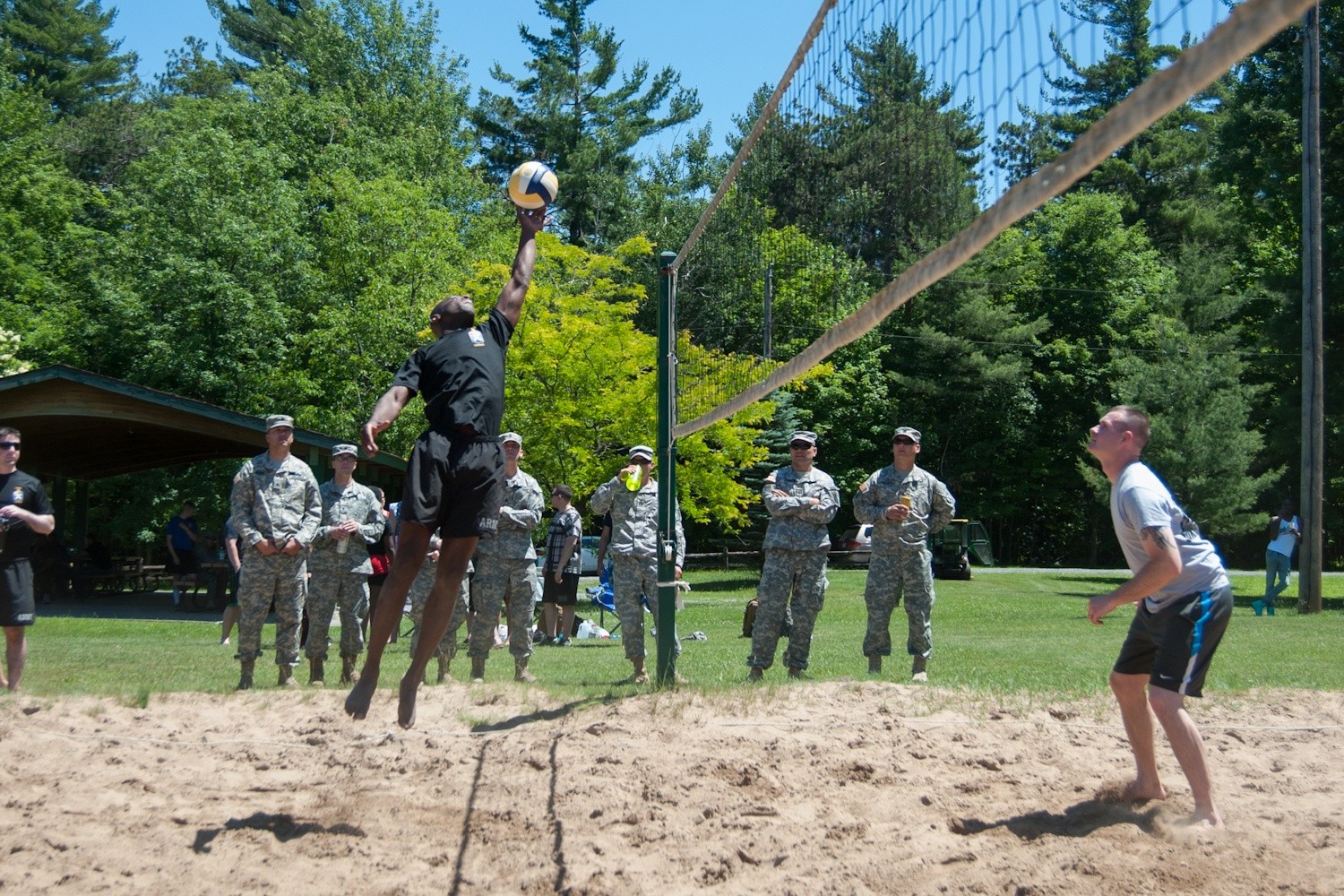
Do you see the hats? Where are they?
[331,443,358,459]
[266,414,294,431]
[629,445,653,461]
[498,432,521,448]
[790,431,817,446]
[892,426,921,444]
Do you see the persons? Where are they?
[230,415,322,690]
[746,431,841,681]
[165,415,545,690]
[852,426,956,682]
[591,445,691,685]
[343,202,555,729]
[1085,404,1234,827]
[596,509,614,576]
[534,485,583,647]
[0,426,113,691]
[1248,499,1303,615]
[304,444,385,685]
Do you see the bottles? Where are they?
[621,465,643,492]
[218,547,226,559]
[336,531,350,553]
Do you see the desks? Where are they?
[77,556,144,594]
[201,562,231,610]
[122,564,166,592]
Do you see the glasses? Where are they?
[0,442,21,451]
[892,438,915,445]
[549,493,565,499]
[630,458,651,465]
[334,455,354,462]
[790,441,812,450]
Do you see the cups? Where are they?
[900,497,910,517]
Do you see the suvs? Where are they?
[926,518,995,581]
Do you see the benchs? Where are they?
[85,572,226,612]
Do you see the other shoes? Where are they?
[539,635,572,646]
[1251,600,1275,616]
[749,668,763,682]
[788,666,813,680]
[221,638,230,644]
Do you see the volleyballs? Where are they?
[509,161,559,209]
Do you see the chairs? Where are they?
[584,563,653,635]
[531,582,544,622]
[397,590,415,637]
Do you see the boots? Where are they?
[513,654,538,683]
[868,654,881,674]
[436,657,452,681]
[469,656,485,683]
[629,657,649,685]
[235,652,361,689]
[911,657,928,683]
[671,669,692,684]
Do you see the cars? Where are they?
[533,533,613,578]
[831,522,875,570]
